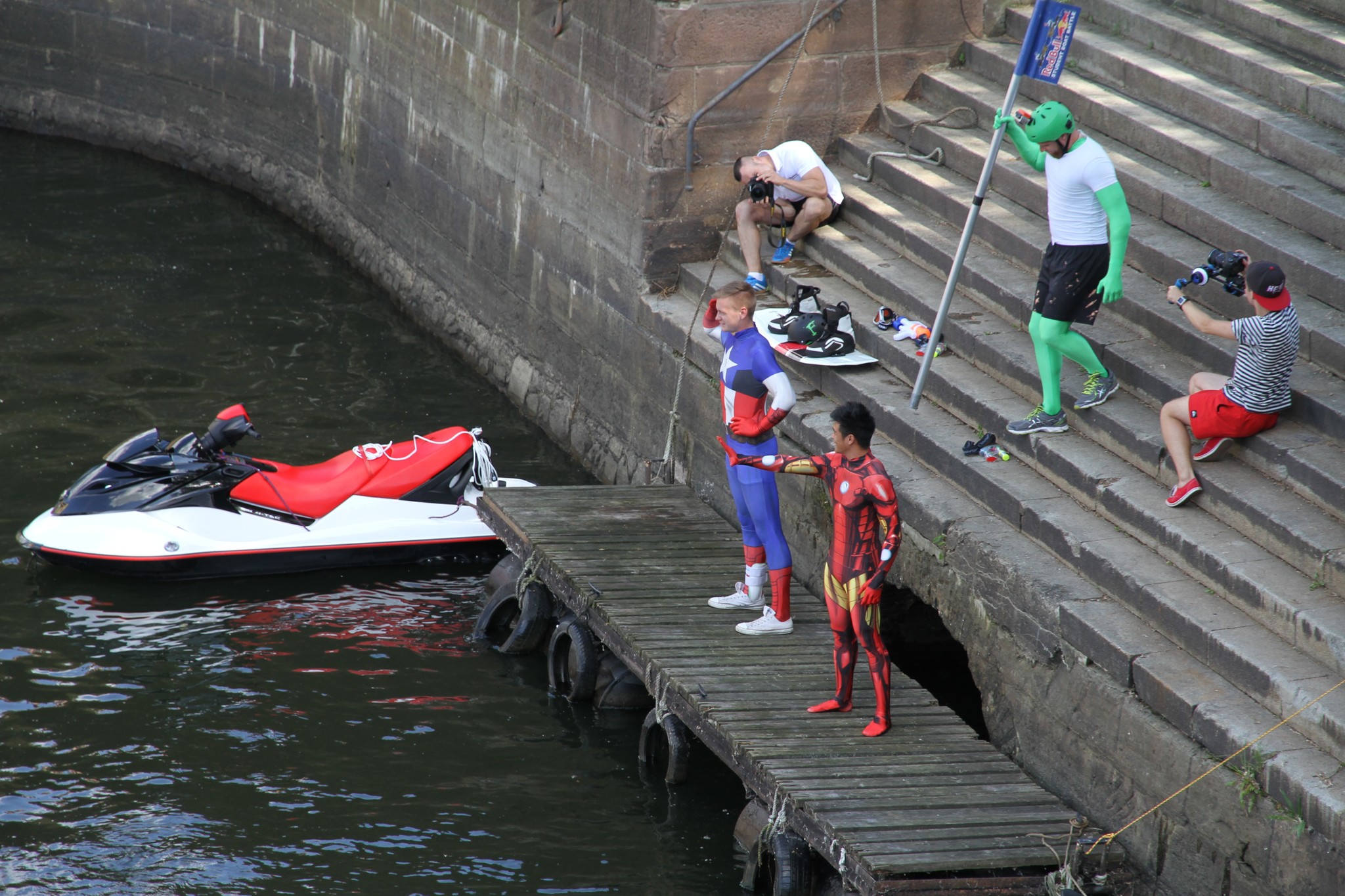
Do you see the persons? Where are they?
[716,402,902,737]
[993,100,1131,435]
[734,141,845,298]
[702,282,794,635]
[1161,250,1300,509]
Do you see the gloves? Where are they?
[1096,271,1124,304]
[993,108,1017,134]
[702,299,720,328]
[857,576,882,607]
[716,435,742,467]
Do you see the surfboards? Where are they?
[752,307,879,367]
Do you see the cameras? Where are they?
[747,176,774,201]
[1015,109,1033,125]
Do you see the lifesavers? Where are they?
[740,825,814,896]
[474,578,552,653]
[639,708,690,785]
[547,621,598,705]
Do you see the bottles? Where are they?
[933,348,941,358]
[998,450,1010,461]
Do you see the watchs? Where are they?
[1177,296,1192,311]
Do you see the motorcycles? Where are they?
[11,400,541,584]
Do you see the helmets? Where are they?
[1025,101,1075,143]
[788,313,826,345]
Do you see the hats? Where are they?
[1246,260,1291,311]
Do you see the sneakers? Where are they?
[1074,367,1119,409]
[743,274,769,294]
[735,605,796,635]
[805,301,856,358]
[1193,437,1234,462]
[768,285,822,334]
[1006,403,1069,435]
[1165,478,1203,507]
[771,239,795,264]
[708,582,766,610]
